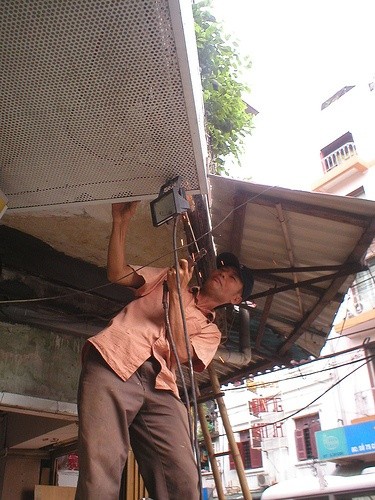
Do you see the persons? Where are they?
[74,189,255,500]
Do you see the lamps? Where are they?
[149,175,190,228]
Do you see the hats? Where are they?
[216,252,254,302]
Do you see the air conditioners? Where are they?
[258,474,271,486]
